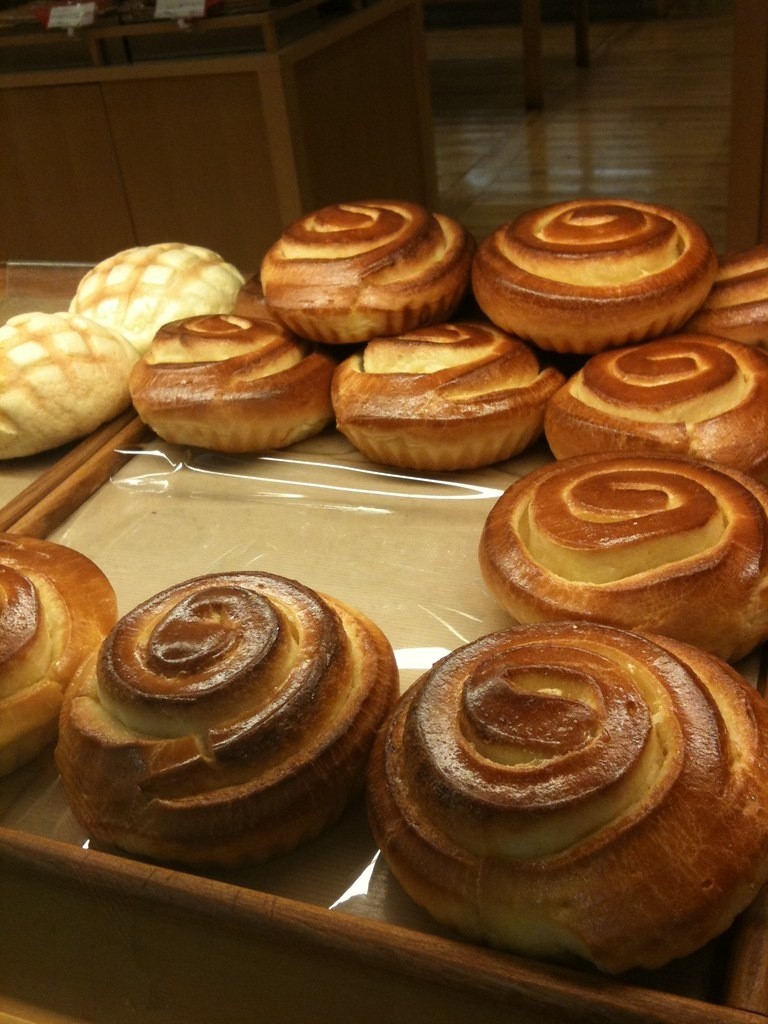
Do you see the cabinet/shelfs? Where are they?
[0,0,440,273]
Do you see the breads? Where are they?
[0,196,768,974]
